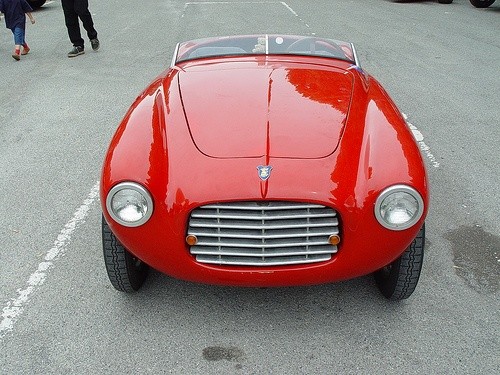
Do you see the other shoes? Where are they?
[21,48,30,55]
[12,55,20,61]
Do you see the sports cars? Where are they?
[100,34,430,301]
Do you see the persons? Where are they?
[0,0,35,61]
[61,0,100,56]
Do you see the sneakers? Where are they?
[68,45,85,56]
[91,38,99,49]
